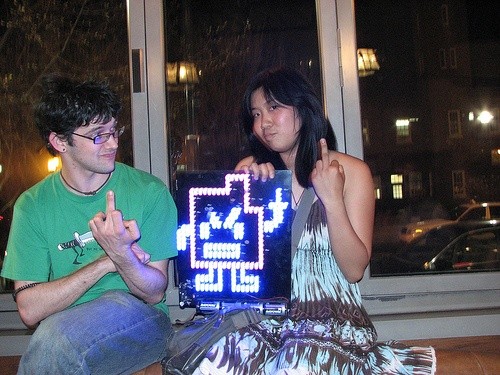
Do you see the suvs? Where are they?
[399,200,500,244]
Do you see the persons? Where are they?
[161,65,376,375]
[0,71,170,375]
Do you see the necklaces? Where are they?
[290,188,306,211]
[58,169,112,195]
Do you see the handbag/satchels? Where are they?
[162,309,267,375]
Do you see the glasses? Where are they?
[71,124,125,144]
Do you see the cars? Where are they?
[377,221,500,273]
